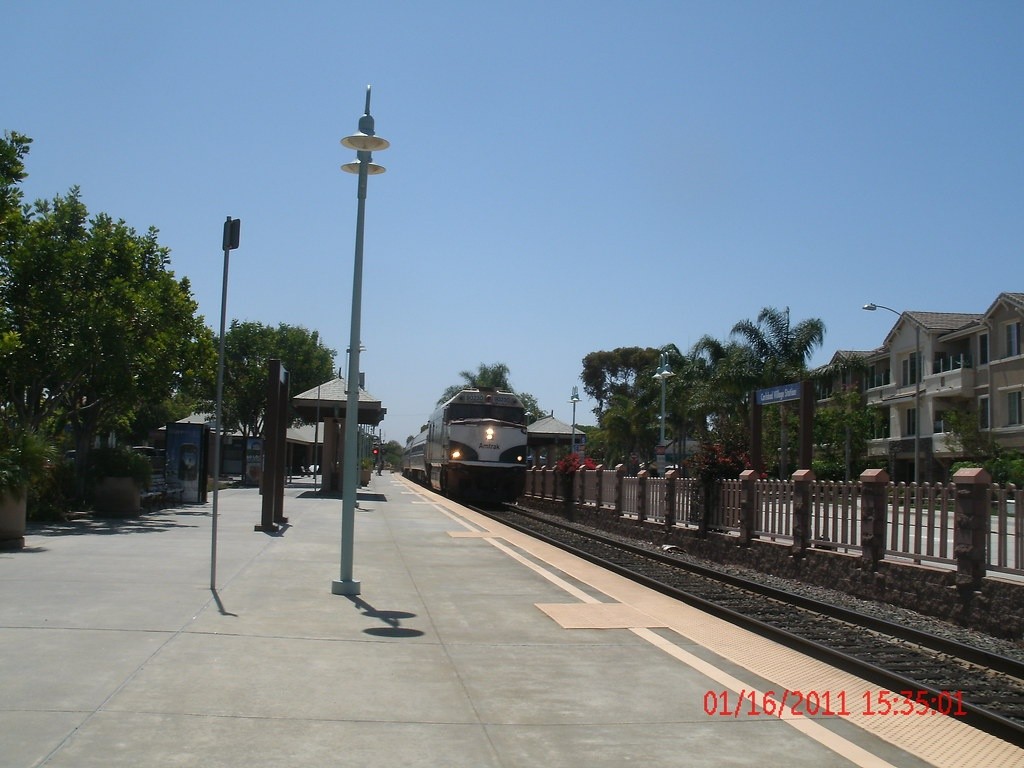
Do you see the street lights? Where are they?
[863,302,921,482]
[340,84,391,581]
[566,384,583,453]
[651,352,676,445]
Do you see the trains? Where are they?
[401,387,533,506]
